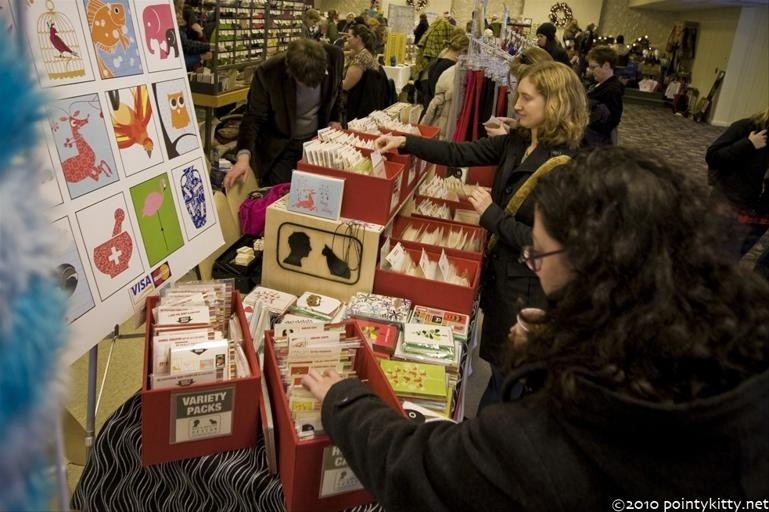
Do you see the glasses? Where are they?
[517,245,571,272]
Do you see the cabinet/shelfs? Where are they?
[175,0,310,165]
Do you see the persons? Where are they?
[173,0,766,373]
[299,140,769,512]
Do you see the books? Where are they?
[148,109,493,440]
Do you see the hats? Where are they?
[536,22,556,40]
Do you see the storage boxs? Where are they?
[142,116,492,512]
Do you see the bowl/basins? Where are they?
[93,230,134,279]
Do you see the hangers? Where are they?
[506,30,535,57]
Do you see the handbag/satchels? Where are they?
[239,183,291,236]
